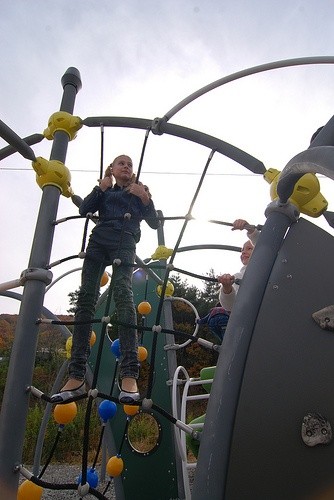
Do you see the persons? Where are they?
[217,219,261,311]
[51,155,159,404]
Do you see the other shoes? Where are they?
[118,382,140,405]
[50,380,86,403]
